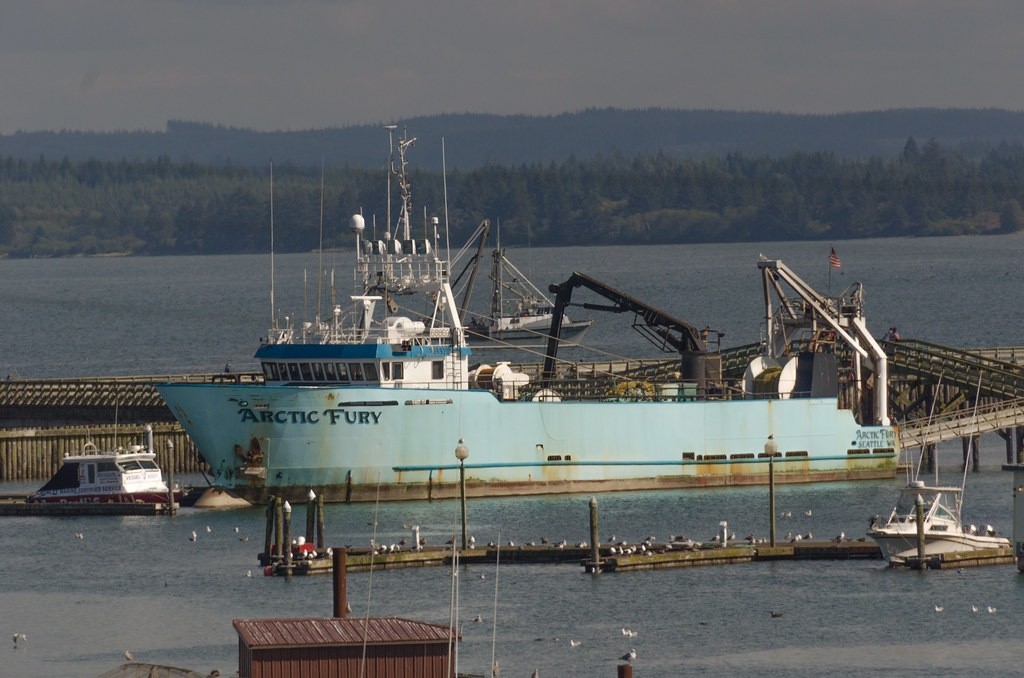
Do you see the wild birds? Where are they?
[12,508,1000,666]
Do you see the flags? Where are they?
[830,247,841,270]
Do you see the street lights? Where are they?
[764,433,778,547]
[454,436,469,551]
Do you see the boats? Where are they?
[149,125,907,507]
[424,216,595,343]
[21,386,194,504]
[866,368,1013,570]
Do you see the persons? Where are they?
[882,326,900,356]
